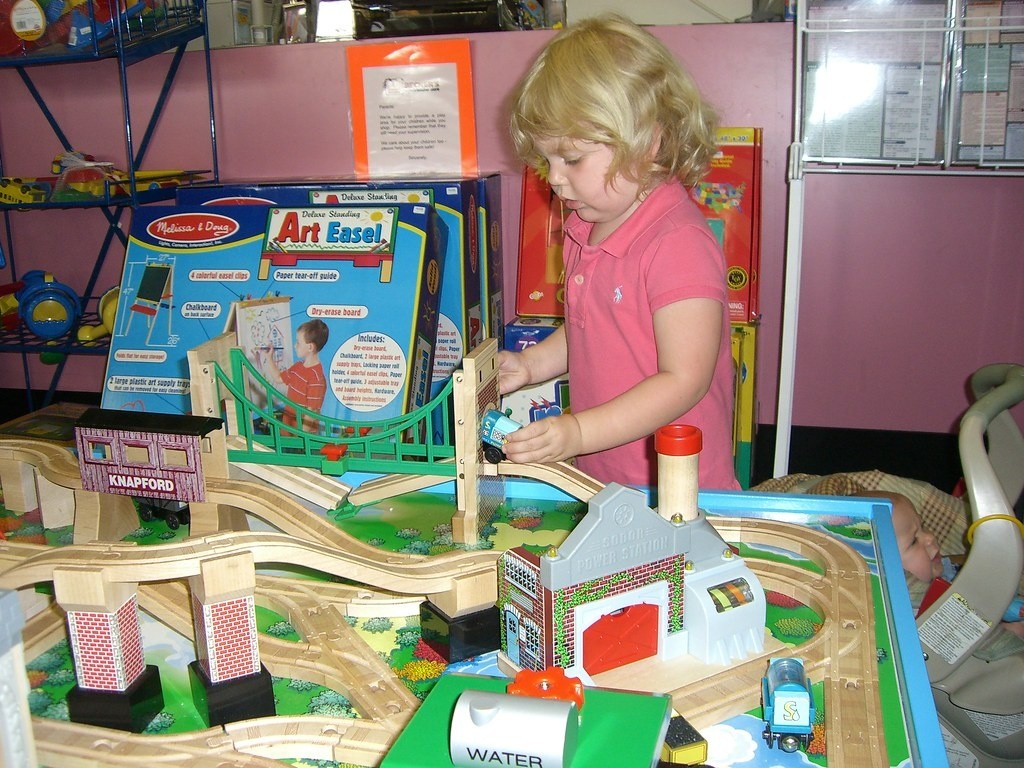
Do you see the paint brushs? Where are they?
[255,346,285,349]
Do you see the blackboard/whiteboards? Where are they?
[223,297,293,412]
[137,263,173,304]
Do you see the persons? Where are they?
[261,319,329,436]
[847,491,1024,638]
[497,11,745,491]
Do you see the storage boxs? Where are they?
[175,174,504,358]
[101,202,449,444]
[504,127,763,491]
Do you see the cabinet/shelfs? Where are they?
[0,0,218,414]
